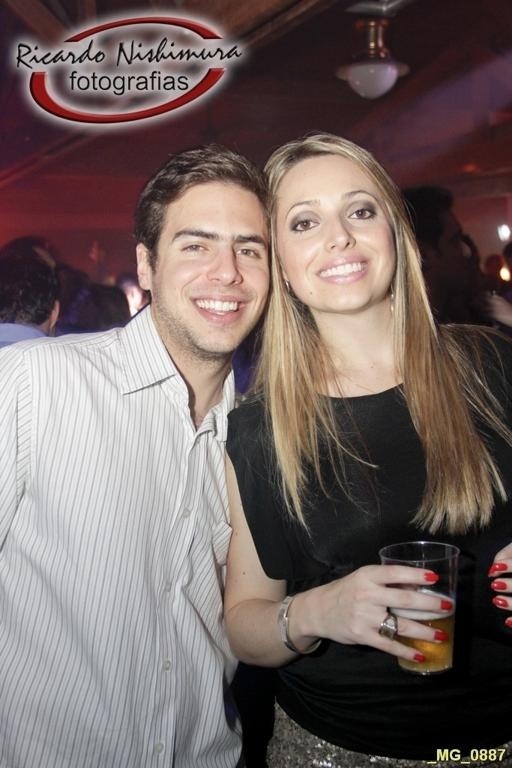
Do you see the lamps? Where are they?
[333,17,411,109]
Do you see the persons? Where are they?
[0,183,512,358]
[2,143,271,768]
[221,133,511,768]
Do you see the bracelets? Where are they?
[275,593,324,655]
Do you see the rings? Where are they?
[375,610,398,639]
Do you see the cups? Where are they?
[377,541,456,677]
